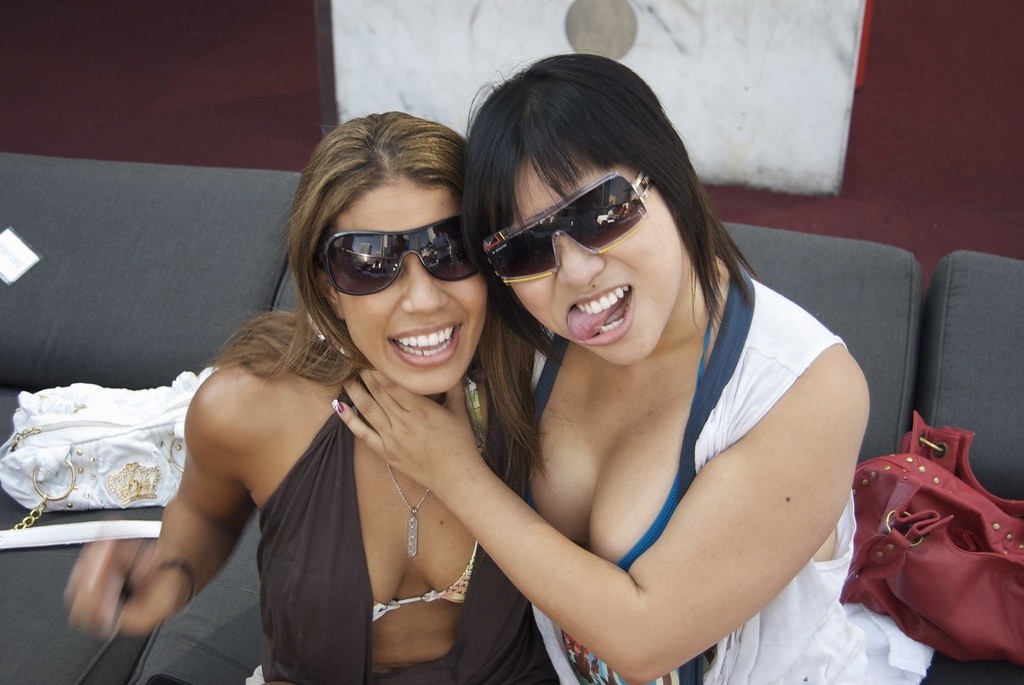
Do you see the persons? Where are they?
[331,55,938,685]
[66,111,561,685]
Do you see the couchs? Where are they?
[0,149,1024,685]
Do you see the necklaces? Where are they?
[387,464,429,558]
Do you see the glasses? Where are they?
[483,171,654,286]
[316,214,481,296]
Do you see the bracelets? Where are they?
[160,559,196,606]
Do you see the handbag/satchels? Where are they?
[0,366,219,549]
[839,410,1024,668]
